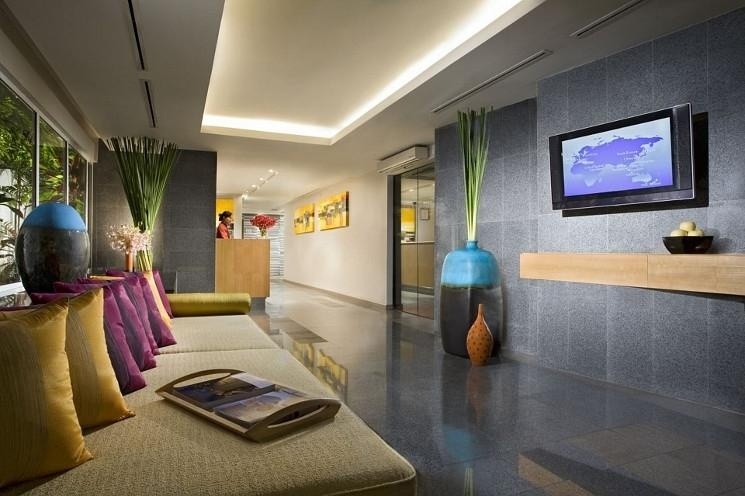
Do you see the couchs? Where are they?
[1,292,416,496]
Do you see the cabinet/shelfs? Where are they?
[401,241,434,296]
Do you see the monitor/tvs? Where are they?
[547,102,695,211]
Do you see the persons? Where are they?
[217,211,232,239]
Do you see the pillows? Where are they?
[54,280,157,370]
[0,296,92,488]
[76,277,160,355]
[89,271,173,328]
[29,284,147,397]
[107,270,176,346]
[106,270,174,318]
[0,288,135,429]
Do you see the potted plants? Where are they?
[439,106,504,359]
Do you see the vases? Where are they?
[14,201,92,302]
[125,252,134,273]
[467,302,494,366]
[260,228,267,237]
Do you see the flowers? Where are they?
[103,223,149,257]
[250,213,277,229]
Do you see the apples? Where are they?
[671,221,704,237]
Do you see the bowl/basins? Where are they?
[662,235,715,253]
